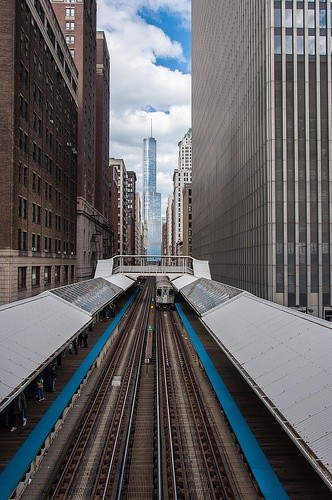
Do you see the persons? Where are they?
[31,298,116,403]
[11,389,28,432]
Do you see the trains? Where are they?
[156,275,174,311]
